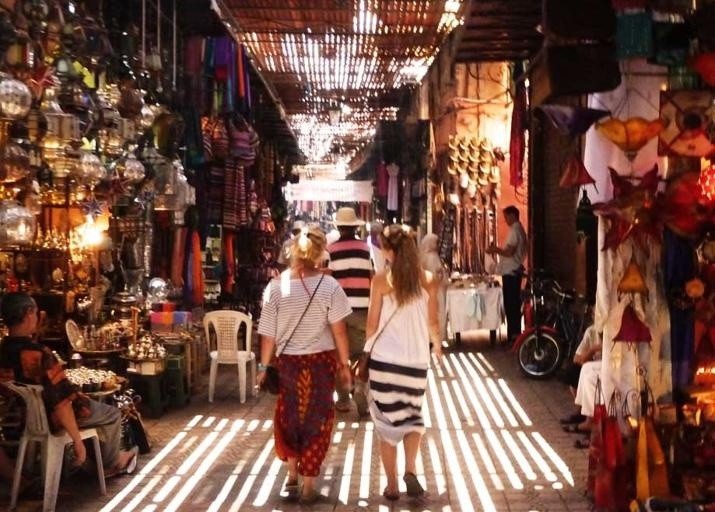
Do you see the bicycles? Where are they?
[512,268,593,388]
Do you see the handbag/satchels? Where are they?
[212,113,260,168]
[258,207,277,236]
[351,352,371,378]
[248,230,280,266]
[264,364,279,393]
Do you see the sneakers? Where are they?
[559,413,591,448]
[403,473,423,495]
[353,389,370,417]
[335,399,353,412]
[383,486,401,500]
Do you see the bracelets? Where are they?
[340,364,349,369]
[257,363,266,372]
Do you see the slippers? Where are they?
[286,484,315,503]
[104,446,139,479]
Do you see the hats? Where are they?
[327,207,366,226]
[292,220,304,229]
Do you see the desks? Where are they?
[445,283,505,349]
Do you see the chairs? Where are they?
[202,309,259,404]
[1,378,108,512]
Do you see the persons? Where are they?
[277,207,445,414]
[486,204,528,347]
[362,224,443,499]
[0,444,70,511]
[254,230,351,501]
[562,305,603,448]
[1,292,140,481]
